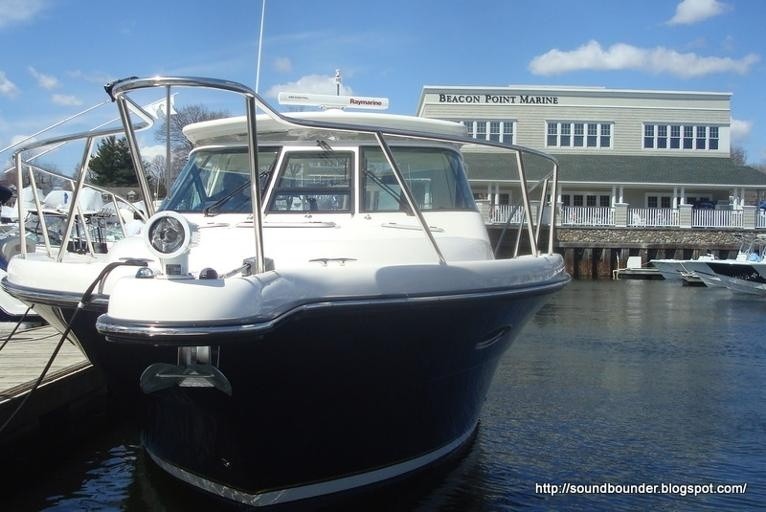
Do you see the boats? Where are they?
[612,236,766,305]
